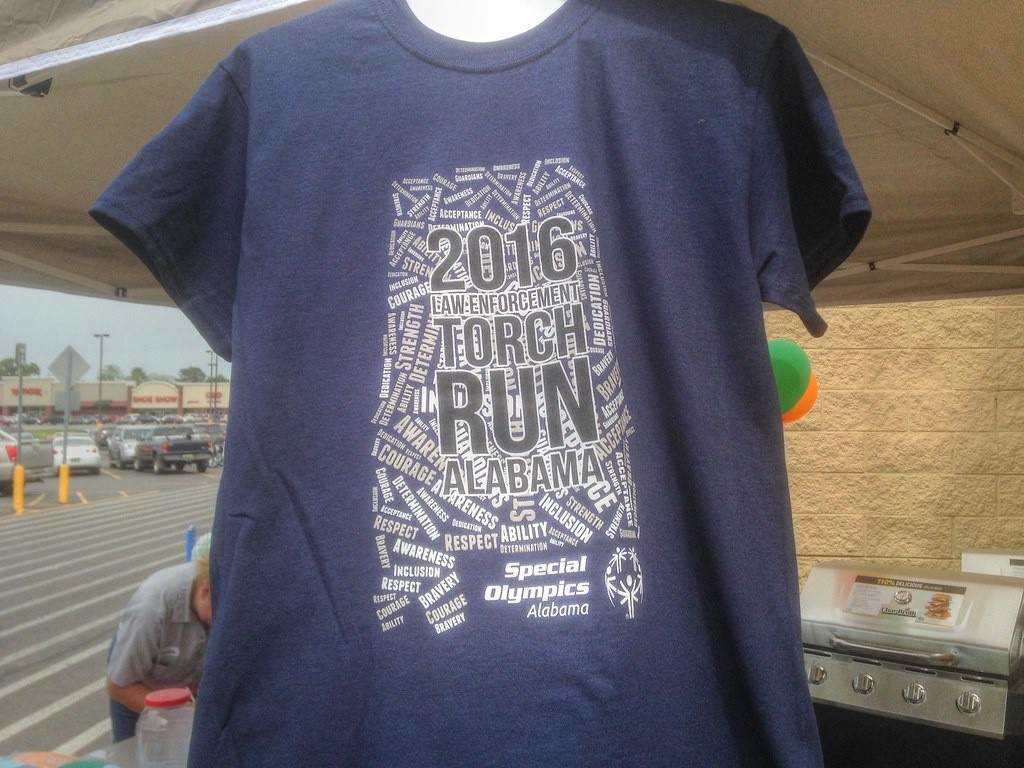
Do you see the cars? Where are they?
[52,436,100,476]
[106,425,156,470]
[0,411,230,428]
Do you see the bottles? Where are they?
[134,688,199,768]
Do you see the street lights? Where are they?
[93,333,109,417]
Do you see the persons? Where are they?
[110,533,214,744]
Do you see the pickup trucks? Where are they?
[0,430,54,489]
[134,425,210,475]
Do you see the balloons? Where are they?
[767,337,819,425]
